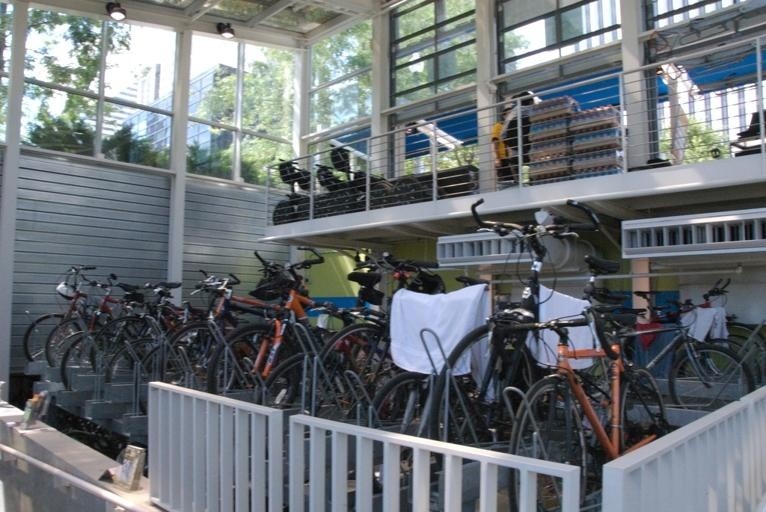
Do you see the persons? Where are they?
[490,91,534,180]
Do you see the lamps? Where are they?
[105,1,126,21]
[217,21,235,39]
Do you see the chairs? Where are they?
[729,109,766,158]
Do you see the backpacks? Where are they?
[507,115,529,153]
